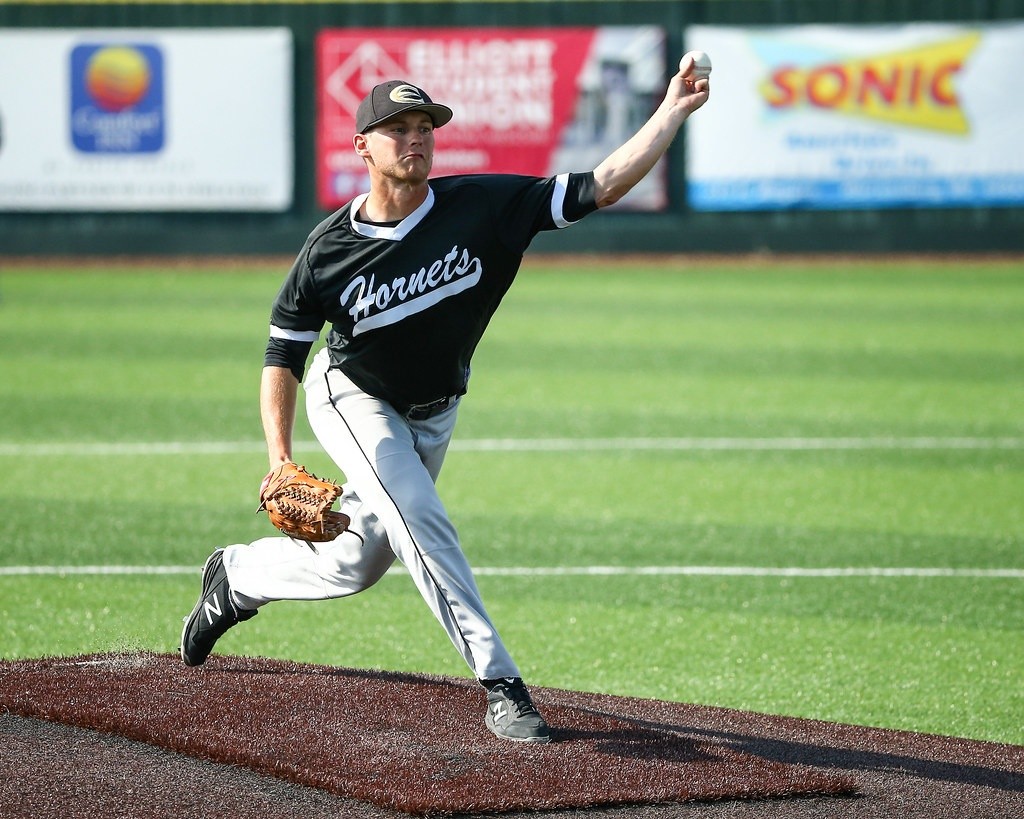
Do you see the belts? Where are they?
[393,392,461,420]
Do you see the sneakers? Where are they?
[484,683,549,743]
[178,547,258,667]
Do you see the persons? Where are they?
[181,57,710,743]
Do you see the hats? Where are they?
[356,80,453,136]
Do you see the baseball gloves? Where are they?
[259,462,350,542]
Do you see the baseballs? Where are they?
[679,51,712,76]
